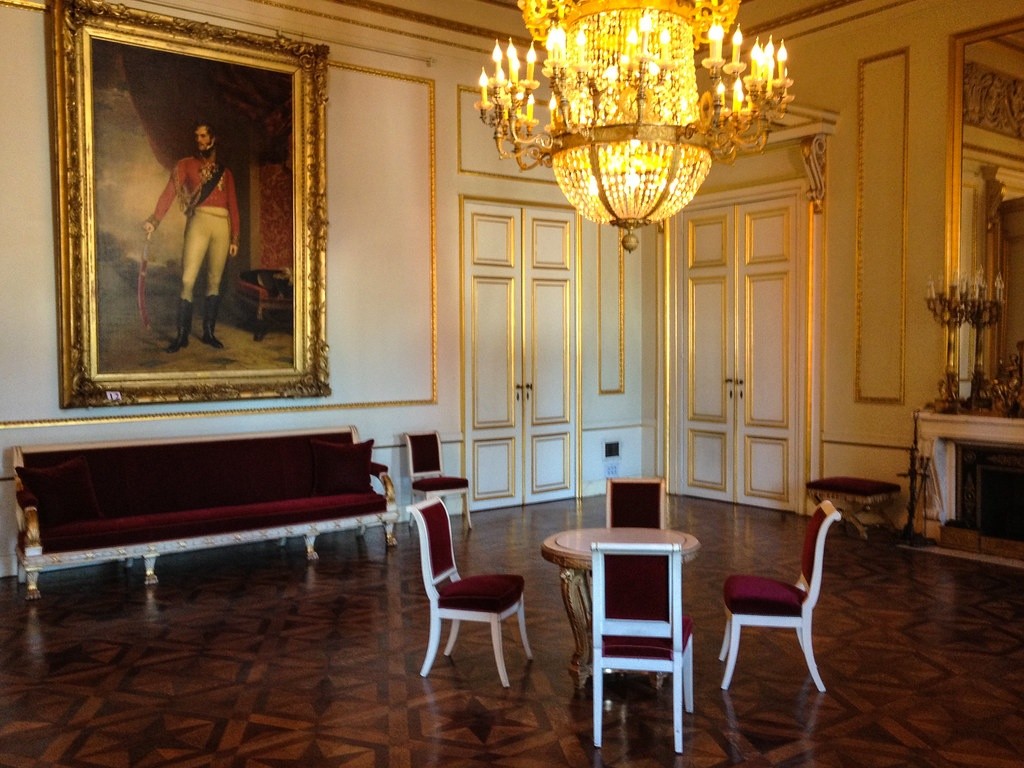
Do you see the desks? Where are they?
[540,526,703,698]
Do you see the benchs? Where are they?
[806,476,903,543]
[10,425,399,599]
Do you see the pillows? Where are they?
[307,435,375,495]
[14,455,105,528]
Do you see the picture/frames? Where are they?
[43,0,332,413]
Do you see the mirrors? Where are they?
[943,15,1024,400]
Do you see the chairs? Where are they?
[403,429,473,530]
[587,541,696,756]
[717,499,843,693]
[604,477,667,529]
[404,495,533,690]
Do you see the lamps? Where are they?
[468,0,796,257]
[956,262,1006,412]
[921,269,975,413]
